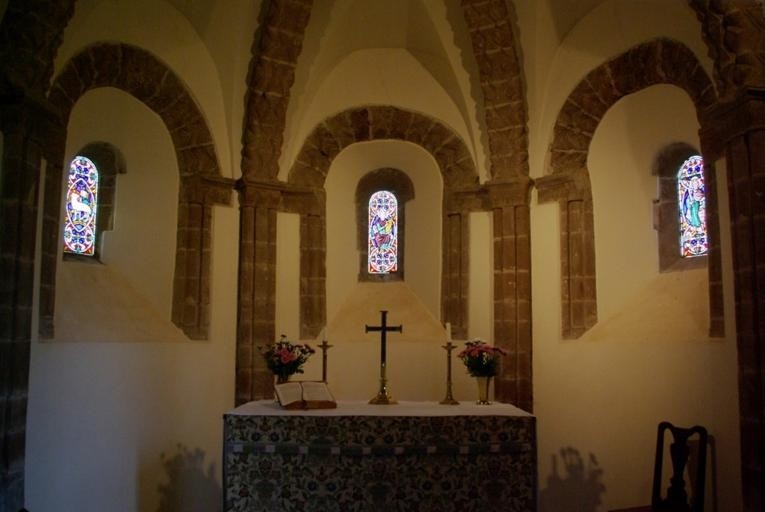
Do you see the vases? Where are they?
[476,376,491,405]
[273,375,289,402]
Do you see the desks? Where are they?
[221,398,538,511]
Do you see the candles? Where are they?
[446,322,452,343]
[321,326,326,341]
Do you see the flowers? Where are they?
[457,339,508,376]
[255,334,315,383]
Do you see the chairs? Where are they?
[610,421,707,512]
